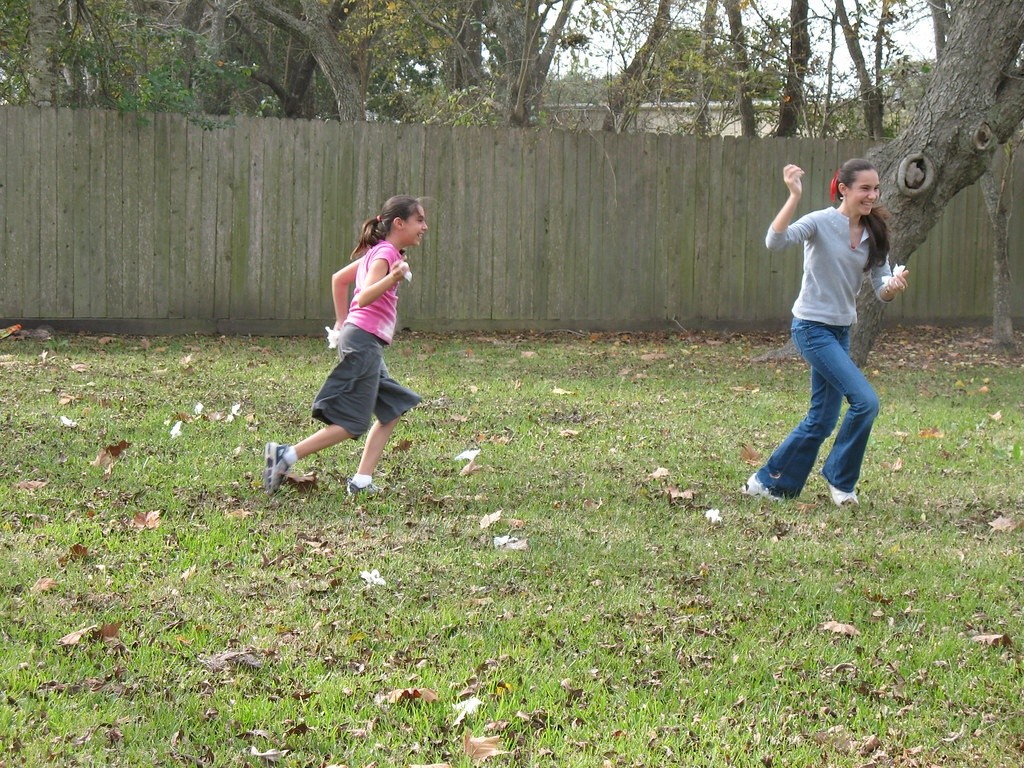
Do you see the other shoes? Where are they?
[819,468,859,509]
[740,473,784,505]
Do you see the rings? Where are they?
[900,286,906,289]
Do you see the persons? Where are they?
[741,157,910,510]
[265,194,428,493]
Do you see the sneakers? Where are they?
[346,477,384,498]
[264,442,293,495]
